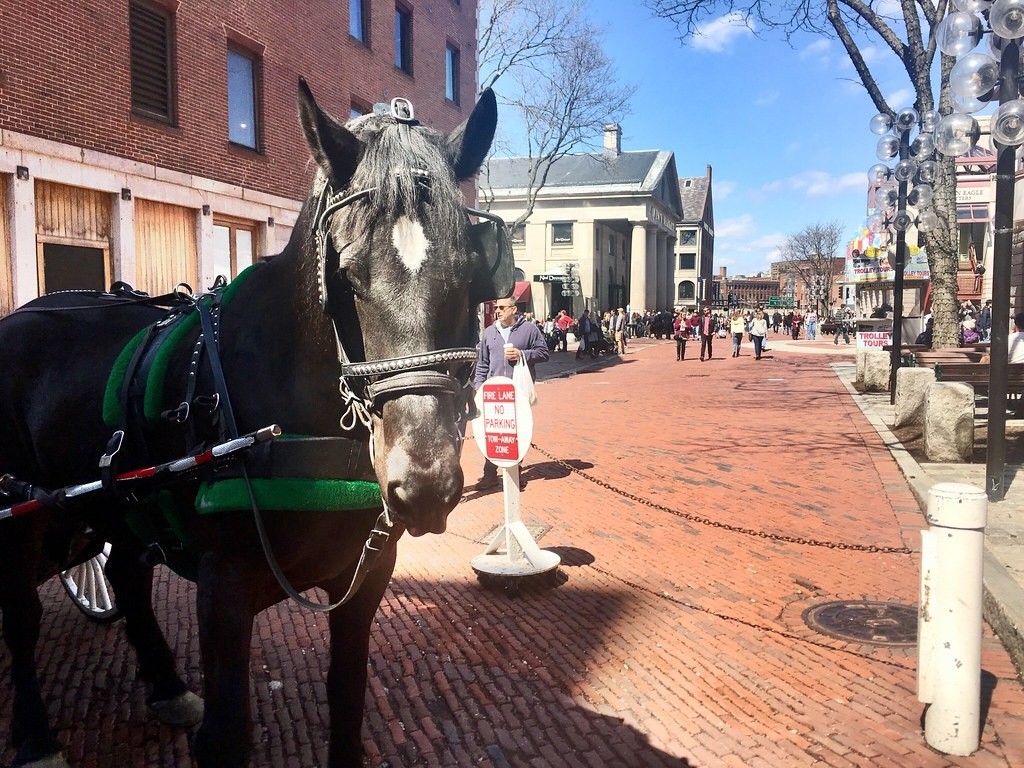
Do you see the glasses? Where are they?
[493,306,514,310]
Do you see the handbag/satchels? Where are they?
[674,334,679,340]
[513,350,537,406]
[580,337,585,350]
[749,334,752,342]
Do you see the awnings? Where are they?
[485,281,530,303]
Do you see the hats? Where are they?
[963,319,976,330]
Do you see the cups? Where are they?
[503,343,513,357]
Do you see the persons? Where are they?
[631,303,893,362]
[521,310,575,352]
[975,261,986,274]
[915,299,1024,364]
[457,309,481,421]
[473,297,550,491]
[575,307,631,360]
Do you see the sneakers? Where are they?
[520,468,527,487]
[476,470,498,489]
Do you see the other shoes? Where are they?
[756,355,761,360]
[700,358,704,361]
[709,354,712,359]
[737,354,740,357]
[677,356,680,361]
[576,355,583,359]
[733,352,736,357]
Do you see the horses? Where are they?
[0,76,499,767]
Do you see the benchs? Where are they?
[883,344,1024,417]
